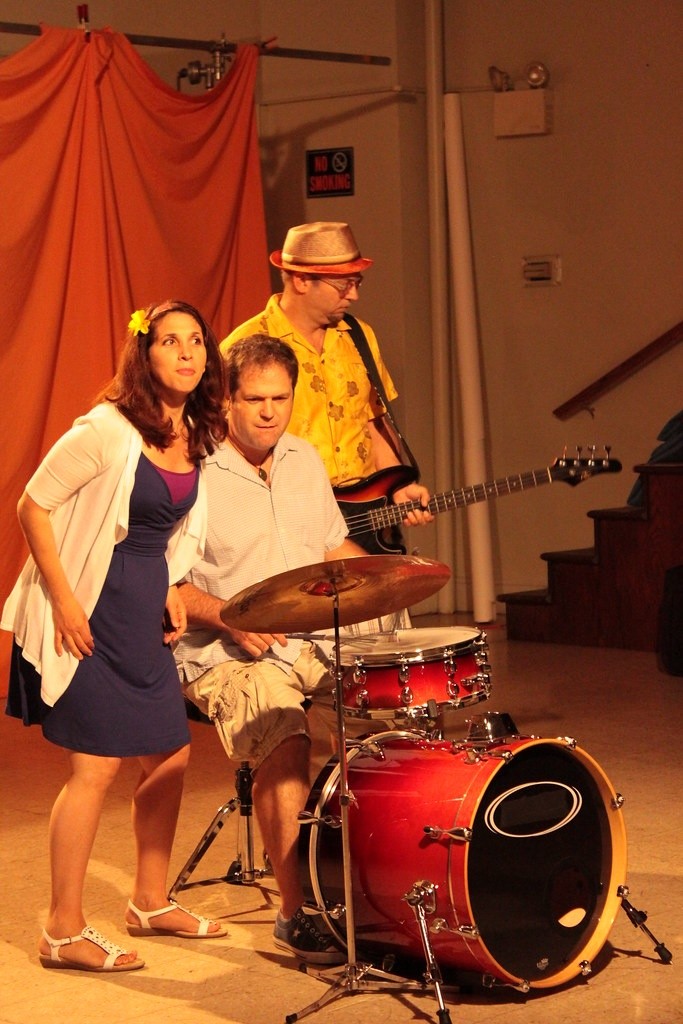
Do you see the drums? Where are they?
[299,727,627,991]
[331,627,492,719]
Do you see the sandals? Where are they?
[40,926,148,971]
[124,898,229,939]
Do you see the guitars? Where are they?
[332,444,622,555]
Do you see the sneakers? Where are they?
[274,910,345,963]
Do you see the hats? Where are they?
[270,221,376,275]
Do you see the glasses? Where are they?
[311,274,366,294]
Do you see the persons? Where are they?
[219,219,435,631]
[1,222,224,972]
[176,332,373,968]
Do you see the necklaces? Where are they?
[228,437,278,481]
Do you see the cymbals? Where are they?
[220,556,451,634]
